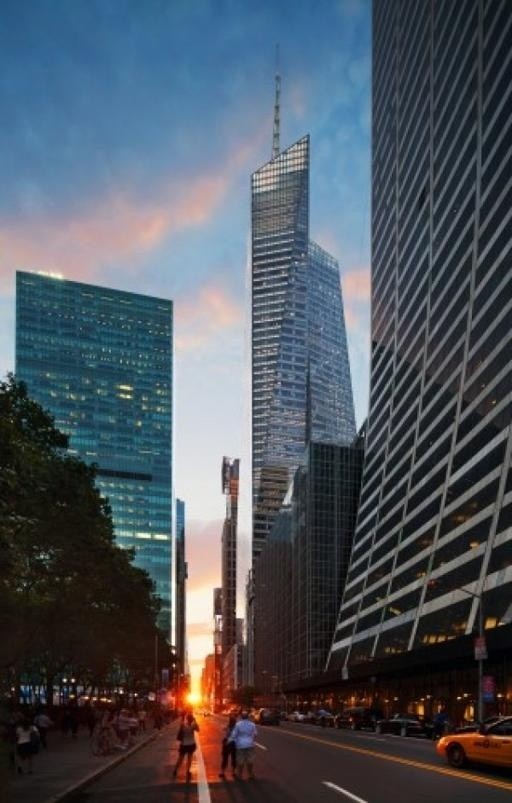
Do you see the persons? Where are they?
[171,714,201,778]
[218,711,257,779]
[1,700,171,777]
[319,707,327,727]
[431,710,445,741]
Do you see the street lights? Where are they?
[427,577,486,722]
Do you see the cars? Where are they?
[454,716,511,734]
[246,704,446,740]
[435,716,512,770]
